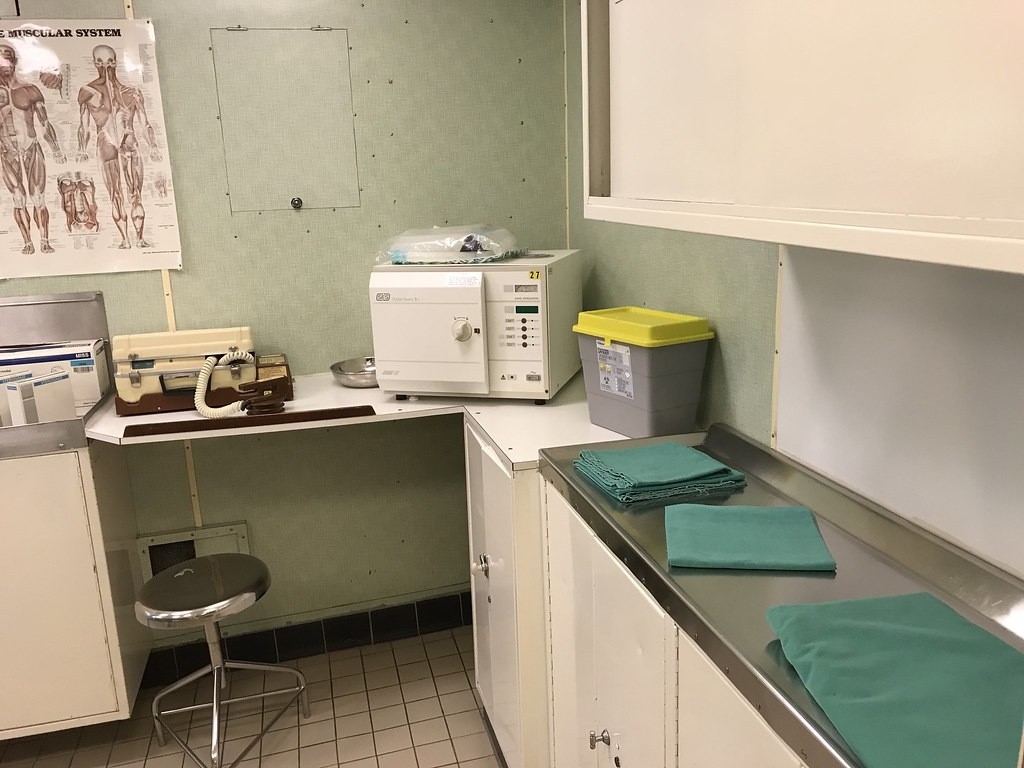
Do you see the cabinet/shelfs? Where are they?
[539,471,809,768]
[466,423,544,768]
[0,443,156,741]
[581,0,1024,275]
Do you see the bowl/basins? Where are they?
[330,357,378,388]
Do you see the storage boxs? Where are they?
[572,306,713,438]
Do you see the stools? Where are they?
[135,553,308,768]
